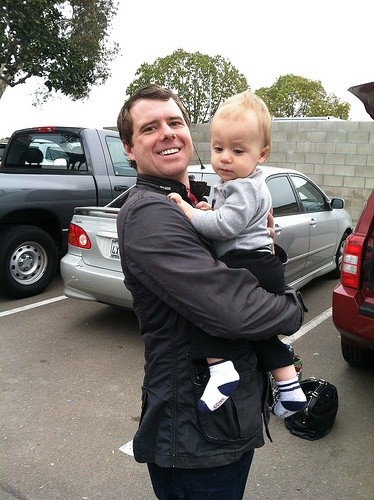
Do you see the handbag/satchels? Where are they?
[284,377,338,441]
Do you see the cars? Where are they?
[61,144,354,310]
[1,138,81,171]
[332,80,374,367]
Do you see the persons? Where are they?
[116,85,308,500]
[167,91,308,419]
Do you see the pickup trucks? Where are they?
[0,128,139,301]
